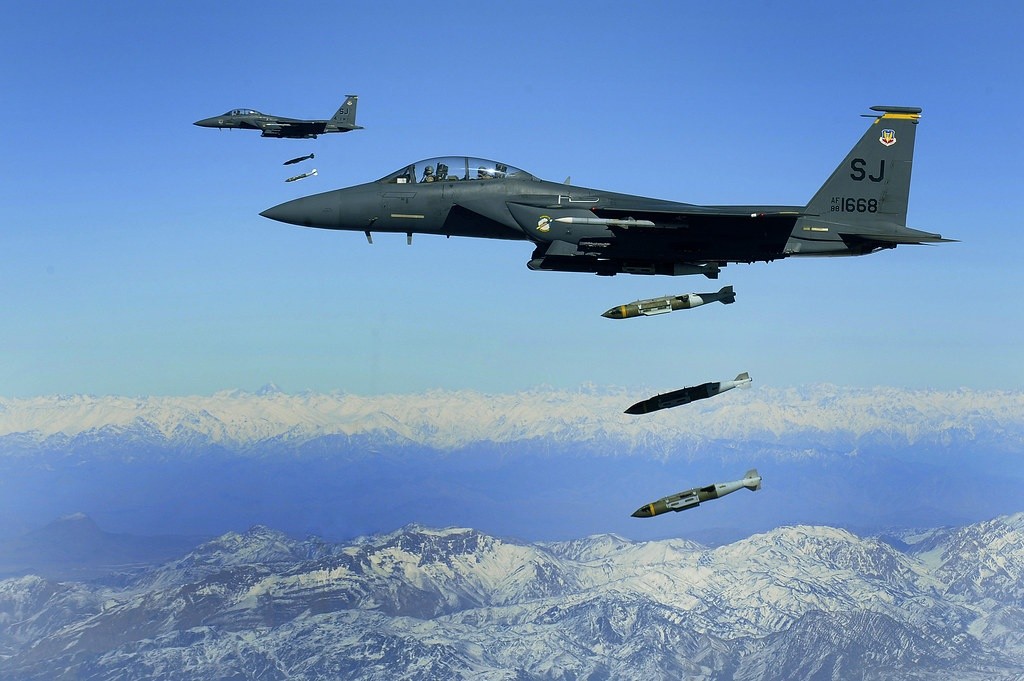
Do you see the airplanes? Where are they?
[258,101,963,281]
[192,95,364,140]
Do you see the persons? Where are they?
[420,166,434,182]
[478,167,491,179]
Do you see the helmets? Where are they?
[423,166,434,174]
[478,166,487,175]
